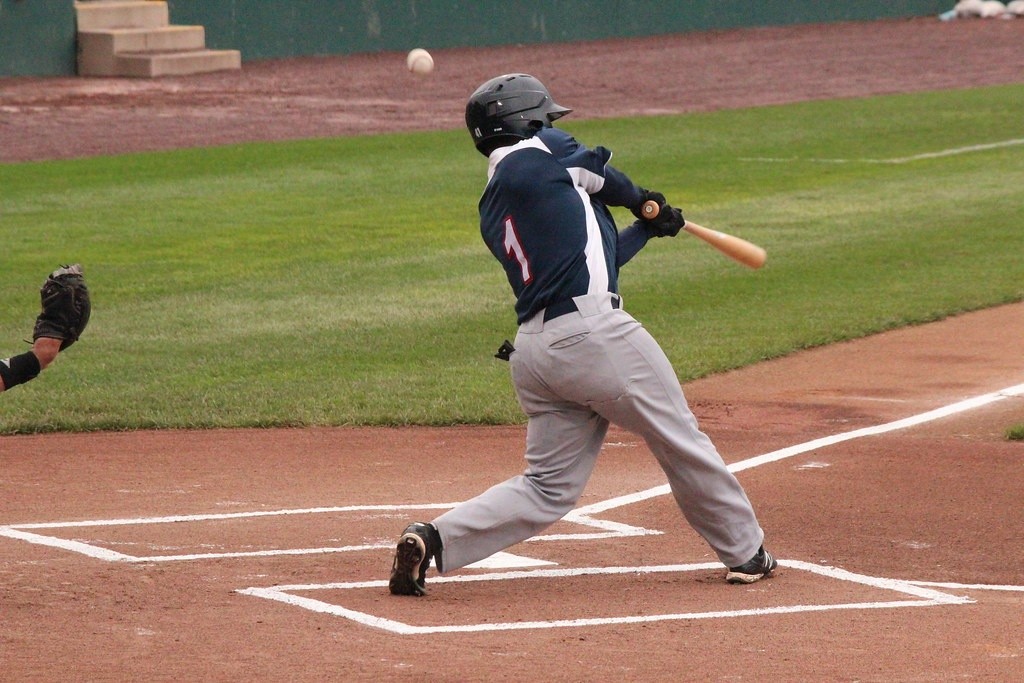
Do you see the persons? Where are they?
[0,262,91,392]
[389,73,780,596]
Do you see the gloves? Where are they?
[634,207,686,238]
[630,188,666,220]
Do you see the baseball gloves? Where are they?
[33,262,91,352]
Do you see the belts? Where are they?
[542,297,620,325]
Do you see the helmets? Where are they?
[465,72,575,158]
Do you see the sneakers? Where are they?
[390,521,433,596]
[725,546,777,584]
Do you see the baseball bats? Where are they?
[642,200,768,271]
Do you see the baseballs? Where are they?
[406,47,435,78]
